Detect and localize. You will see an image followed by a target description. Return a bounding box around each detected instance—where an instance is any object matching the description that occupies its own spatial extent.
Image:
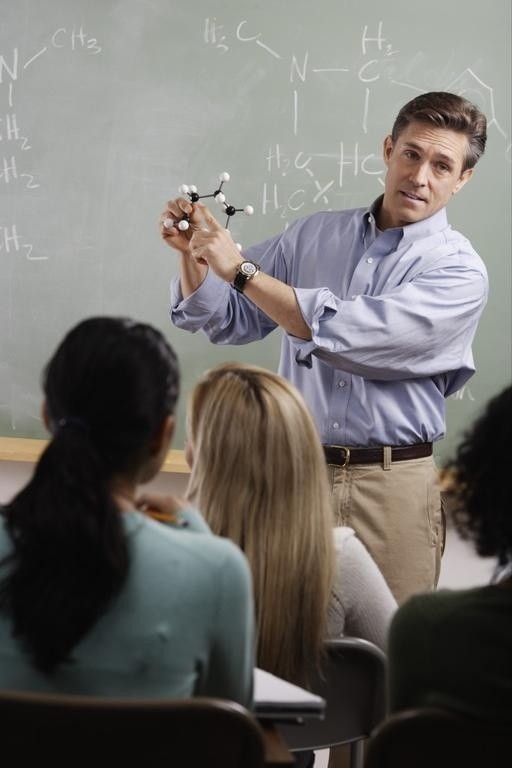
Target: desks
[252,665,327,724]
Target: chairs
[262,634,391,767]
[0,682,269,768]
[359,706,511,767]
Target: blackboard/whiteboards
[0,0,512,474]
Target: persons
[160,92,487,607]
[1,314,255,709]
[185,362,400,692]
[387,382,512,734]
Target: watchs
[233,258,260,293]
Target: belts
[321,442,433,469]
